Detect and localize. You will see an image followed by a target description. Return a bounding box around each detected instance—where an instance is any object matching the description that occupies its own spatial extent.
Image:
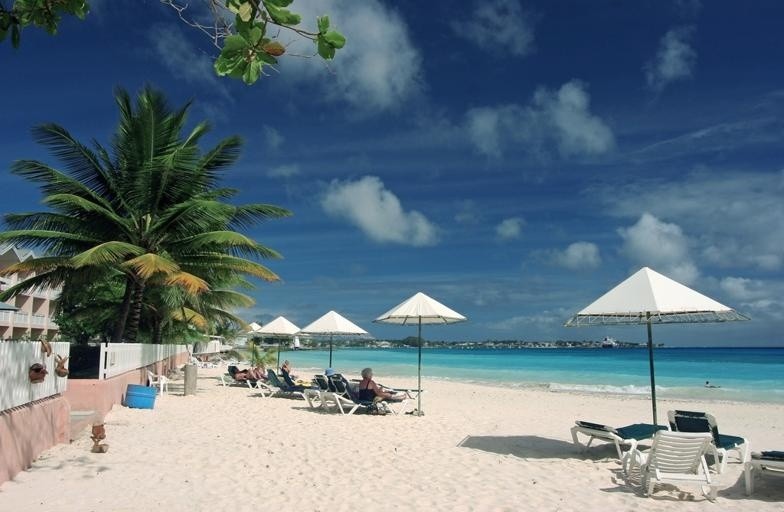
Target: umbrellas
[371,291,467,411]
[250,316,301,375]
[236,321,261,371]
[564,267,752,438]
[299,310,368,367]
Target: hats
[325,369,336,377]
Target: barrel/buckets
[125,384,157,409]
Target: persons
[281,360,290,374]
[231,366,269,382]
[325,368,360,403]
[359,368,406,406]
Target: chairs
[144,353,426,417]
[569,405,784,504]
